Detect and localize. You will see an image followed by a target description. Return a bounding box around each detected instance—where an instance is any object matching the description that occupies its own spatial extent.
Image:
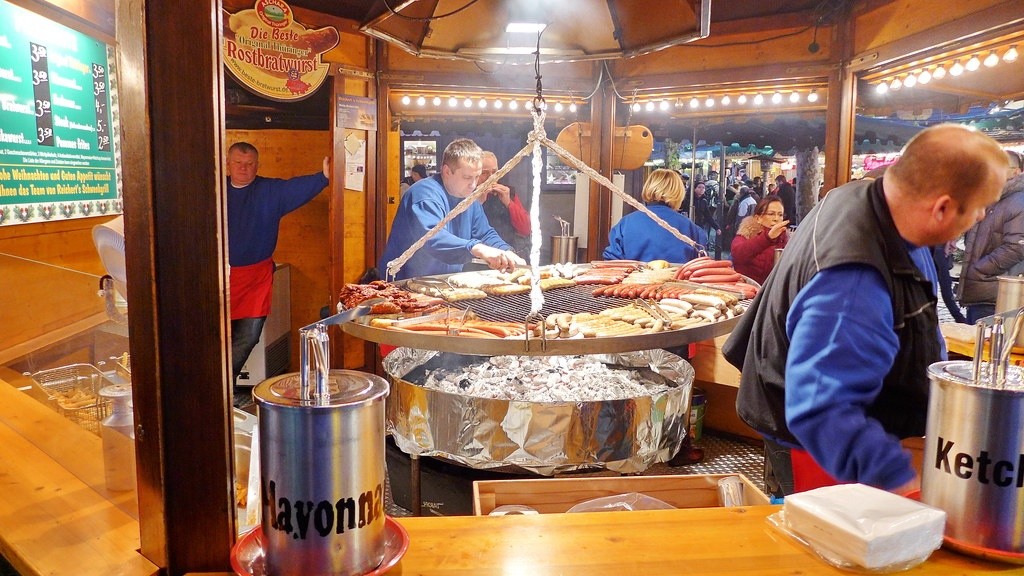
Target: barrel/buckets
[688,386,707,444]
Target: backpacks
[705,184,717,208]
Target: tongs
[446,304,470,337]
[635,297,672,327]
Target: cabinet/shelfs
[547,155,577,185]
[405,152,436,171]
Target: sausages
[369,256,759,340]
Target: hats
[413,166,428,178]
[682,173,689,178]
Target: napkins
[783,483,945,569]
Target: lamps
[505,6,548,35]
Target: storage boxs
[472,474,772,517]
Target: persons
[720,125,1010,502]
[681,173,690,187]
[768,175,795,225]
[404,165,427,186]
[957,149,1024,322]
[381,139,526,281]
[730,195,789,284]
[930,240,963,321]
[680,183,721,236]
[602,169,706,467]
[477,150,529,245]
[695,168,764,249]
[226,142,330,404]
